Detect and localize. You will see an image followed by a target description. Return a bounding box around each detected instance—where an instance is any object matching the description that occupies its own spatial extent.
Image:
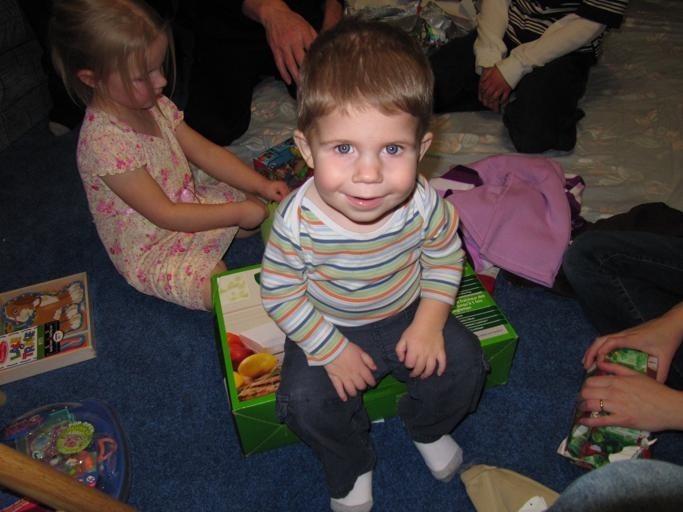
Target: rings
[599,400,606,413]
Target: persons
[340,0,499,119]
[423,0,629,155]
[258,15,492,510]
[151,0,327,150]
[64,0,291,319]
[539,301,683,511]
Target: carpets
[0,119,681,512]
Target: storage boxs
[211,261,520,458]
